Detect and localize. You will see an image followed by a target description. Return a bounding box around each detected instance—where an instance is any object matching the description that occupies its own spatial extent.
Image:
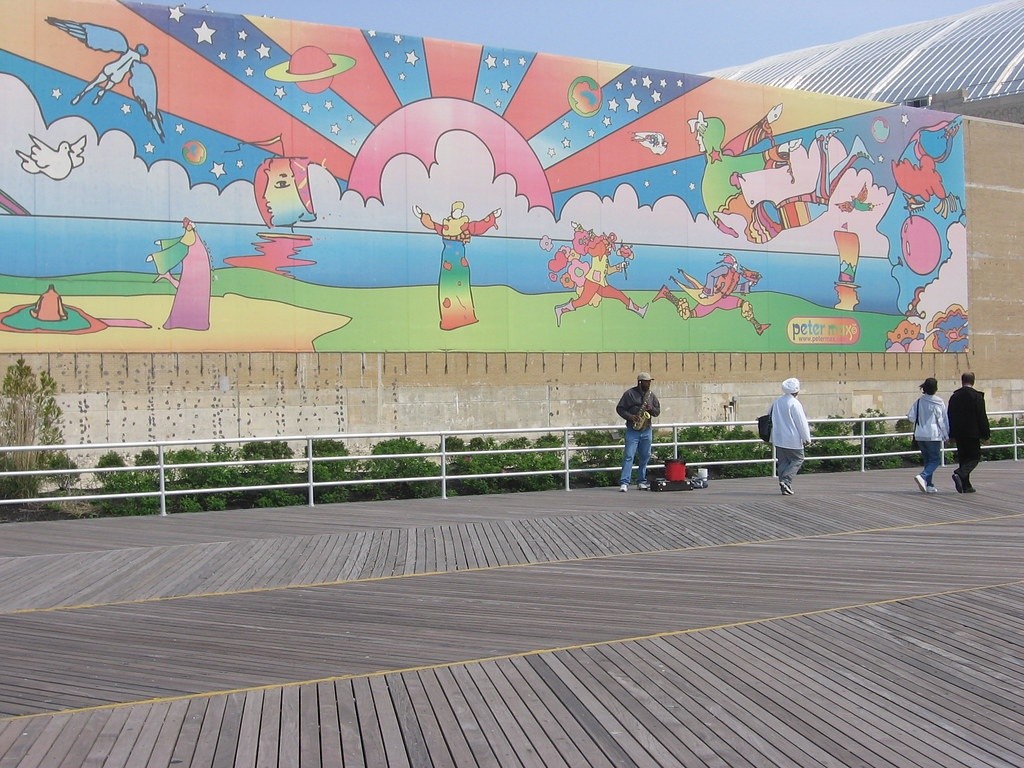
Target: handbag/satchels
[911,434,921,451]
[758,402,773,443]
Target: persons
[616,372,660,492]
[768,378,812,495]
[908,377,950,494]
[947,372,990,493]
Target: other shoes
[779,482,795,495]
[915,475,928,492]
[927,487,937,493]
[620,484,628,492]
[963,487,976,493]
[952,474,963,493]
[639,483,647,489]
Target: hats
[781,378,800,393]
[637,372,655,381]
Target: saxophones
[631,387,652,431]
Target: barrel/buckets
[697,468,709,482]
